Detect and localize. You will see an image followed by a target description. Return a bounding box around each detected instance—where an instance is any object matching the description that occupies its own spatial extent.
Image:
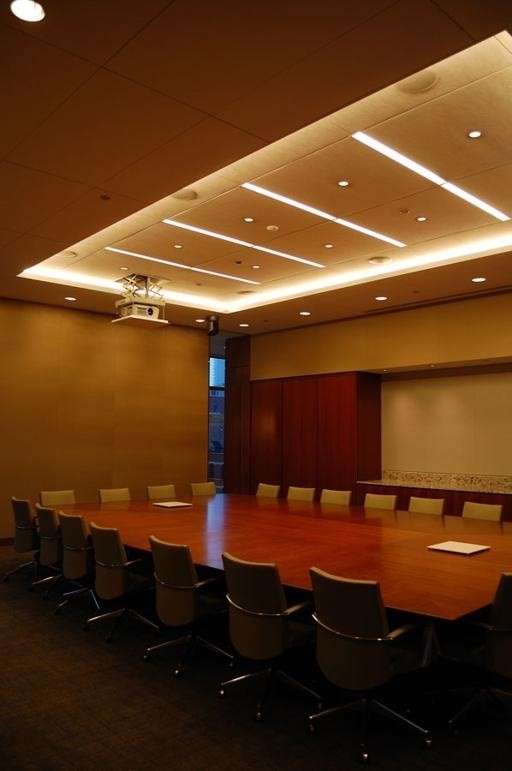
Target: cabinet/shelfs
[319,371,382,504]
[250,378,283,497]
[283,374,319,499]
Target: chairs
[447,573,512,739]
[29,502,63,592]
[53,511,101,615]
[305,567,432,761]
[2,495,41,583]
[143,535,235,678]
[81,522,160,643]
[216,552,324,722]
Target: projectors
[112,298,169,331]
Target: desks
[32,492,512,622]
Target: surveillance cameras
[206,316,219,337]
